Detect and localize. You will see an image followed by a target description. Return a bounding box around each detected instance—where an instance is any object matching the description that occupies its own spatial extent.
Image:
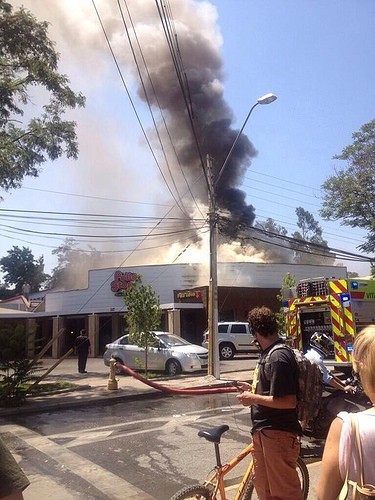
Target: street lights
[205,93,278,379]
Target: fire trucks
[282,276,375,377]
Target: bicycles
[170,425,309,500]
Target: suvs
[201,322,260,360]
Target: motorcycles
[299,375,374,439]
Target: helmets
[309,332,335,358]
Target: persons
[315,325,375,500]
[236,306,304,500]
[22,281,31,302]
[304,332,360,413]
[74,329,92,373]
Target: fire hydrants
[108,355,118,390]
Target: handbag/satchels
[336,411,375,500]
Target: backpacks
[265,343,327,436]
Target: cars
[103,331,209,376]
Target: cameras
[232,380,244,392]
